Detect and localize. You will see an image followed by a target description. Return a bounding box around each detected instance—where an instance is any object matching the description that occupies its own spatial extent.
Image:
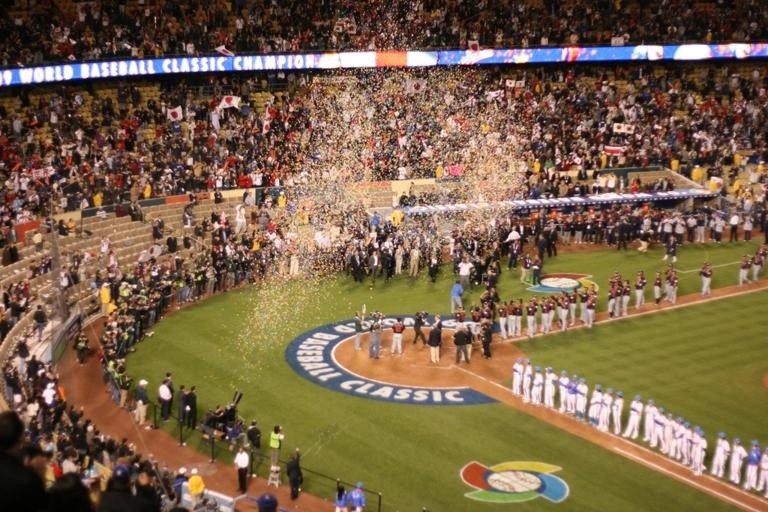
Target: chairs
[1,2,766,417]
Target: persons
[0,2,768,512]
